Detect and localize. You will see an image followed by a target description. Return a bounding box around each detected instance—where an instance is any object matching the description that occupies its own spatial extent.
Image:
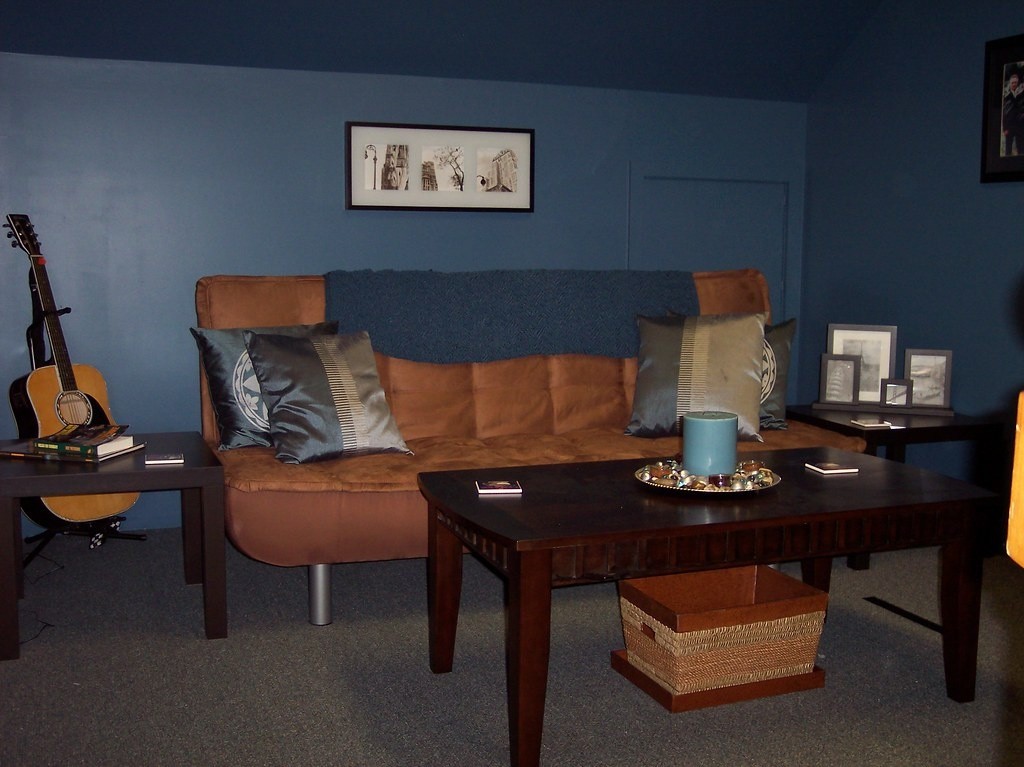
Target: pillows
[624,314,766,445]
[242,329,415,465]
[755,318,803,430]
[189,321,341,451]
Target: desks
[415,446,999,766]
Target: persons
[1002,74,1024,156]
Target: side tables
[0,431,230,661]
[785,402,998,570]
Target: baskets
[619,566,829,693]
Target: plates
[634,467,781,492]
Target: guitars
[3,212,139,533]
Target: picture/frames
[880,377,913,408]
[344,121,535,213]
[980,36,1024,183]
[826,323,898,404]
[903,348,952,409]
[818,353,861,405]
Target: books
[0,422,185,465]
[805,461,860,474]
[475,480,523,494]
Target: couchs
[193,265,869,626]
